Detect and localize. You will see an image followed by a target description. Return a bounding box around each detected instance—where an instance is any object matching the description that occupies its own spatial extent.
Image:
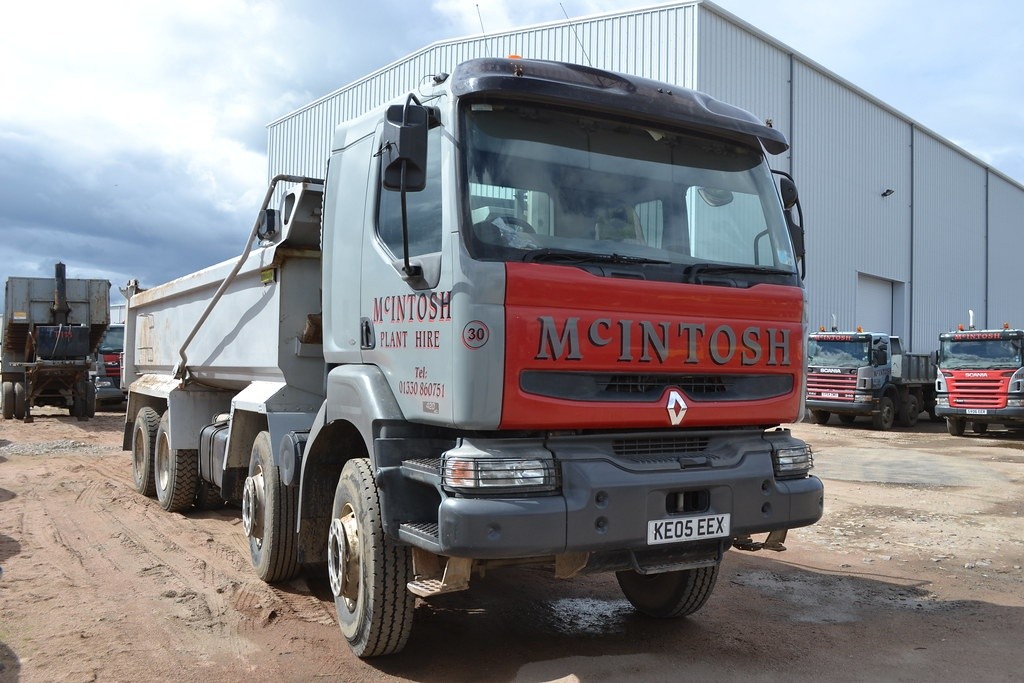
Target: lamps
[881,189,894,197]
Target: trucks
[91,323,132,410]
[120,56,825,660]
[931,309,1024,436]
[805,326,939,430]
